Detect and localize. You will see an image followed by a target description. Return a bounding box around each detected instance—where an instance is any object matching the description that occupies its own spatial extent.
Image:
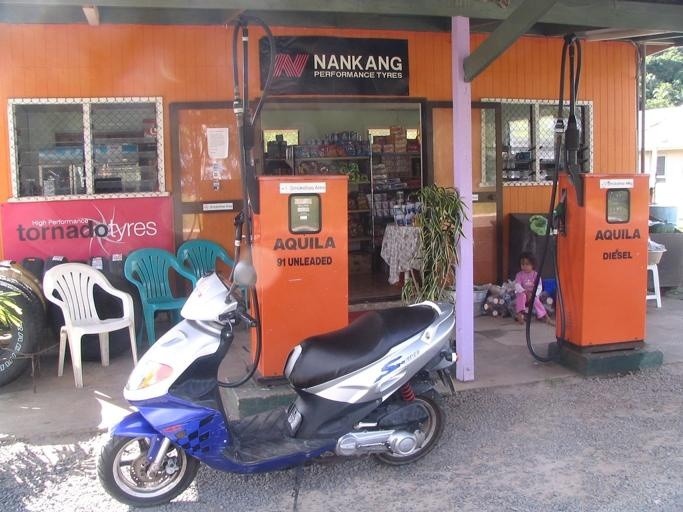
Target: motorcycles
[98,260,456,507]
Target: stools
[646,264,661,308]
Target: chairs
[124,248,197,353]
[42,262,138,388]
[176,237,248,322]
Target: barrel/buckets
[649,205,677,228]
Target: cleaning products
[393,202,420,226]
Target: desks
[509,213,555,282]
[380,223,424,285]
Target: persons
[507,253,555,327]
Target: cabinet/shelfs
[265,133,421,254]
